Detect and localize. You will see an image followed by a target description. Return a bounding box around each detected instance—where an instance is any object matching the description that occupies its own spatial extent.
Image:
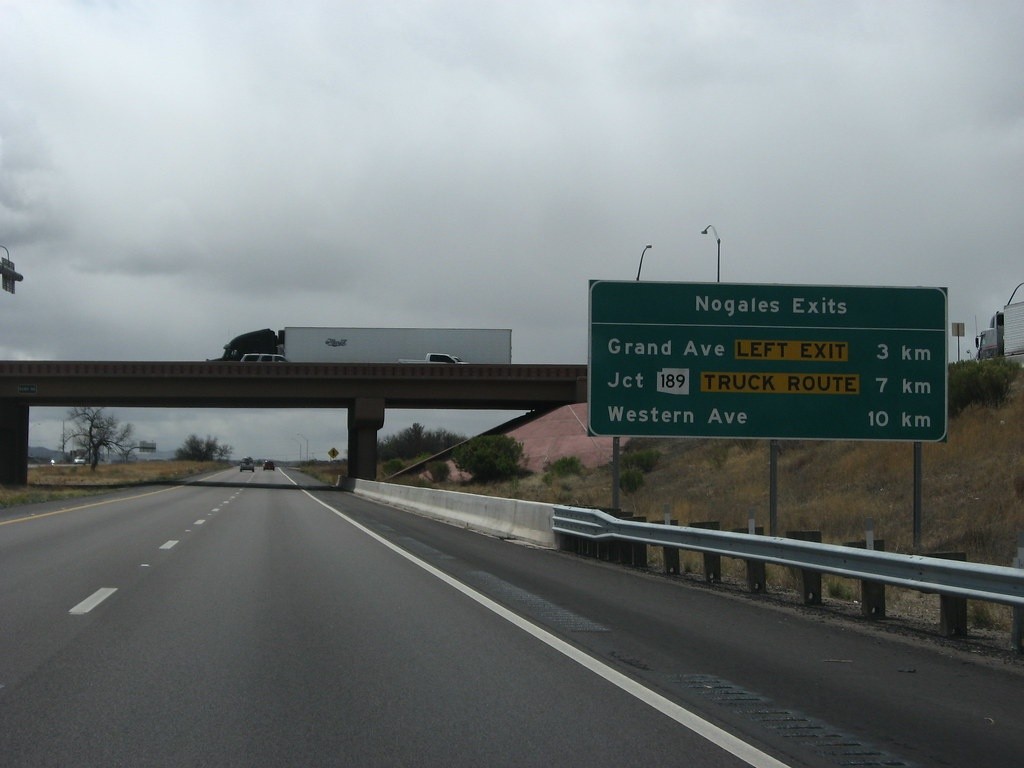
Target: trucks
[975,302,1024,358]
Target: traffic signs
[583,280,947,442]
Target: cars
[263,459,274,471]
[240,456,255,473]
[75,456,86,464]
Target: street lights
[292,438,301,460]
[297,433,308,461]
[610,245,653,282]
[702,225,720,281]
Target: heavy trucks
[202,328,513,364]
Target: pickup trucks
[398,352,470,364]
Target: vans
[241,353,289,363]
